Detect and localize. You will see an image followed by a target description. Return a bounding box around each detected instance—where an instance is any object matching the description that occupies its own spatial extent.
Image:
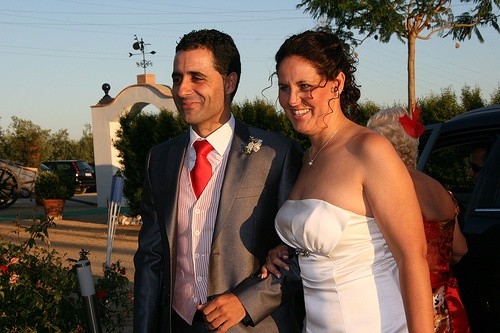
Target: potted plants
[33,173,76,220]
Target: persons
[261,31,434,333]
[366,102,471,333]
[133,29,304,333]
[467,140,494,185]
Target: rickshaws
[0,159,98,211]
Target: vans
[42,160,96,194]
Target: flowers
[245,136,262,152]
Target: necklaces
[308,118,347,166]
